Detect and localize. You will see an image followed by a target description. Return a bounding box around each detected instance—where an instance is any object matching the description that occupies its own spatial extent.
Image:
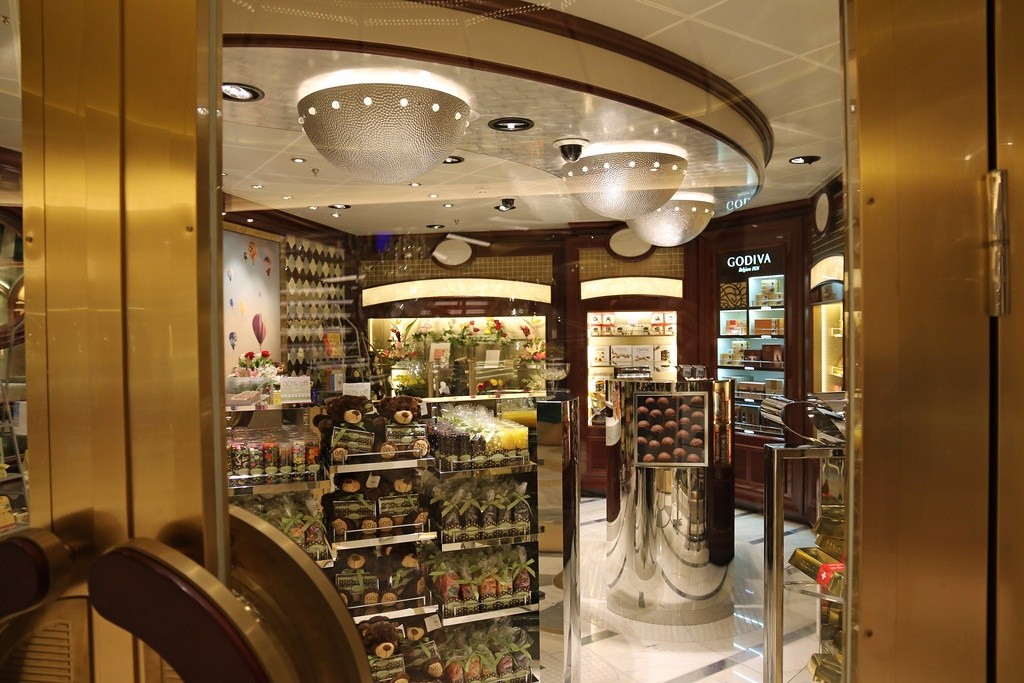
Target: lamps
[556,139,689,220]
[431,238,475,270]
[625,189,716,247]
[810,186,835,237]
[293,68,474,186]
[604,225,657,263]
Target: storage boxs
[726,279,784,426]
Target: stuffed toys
[396,613,444,683]
[373,469,429,532]
[332,547,380,609]
[357,616,410,683]
[377,543,427,607]
[317,395,378,462]
[374,393,431,457]
[321,471,379,534]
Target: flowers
[412,320,432,341]
[488,319,509,345]
[518,319,548,365]
[440,319,457,342]
[457,320,480,345]
[388,318,416,352]
[238,350,271,370]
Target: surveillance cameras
[501,198,514,208]
[553,138,590,163]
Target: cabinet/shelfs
[699,246,803,527]
[221,278,682,682]
